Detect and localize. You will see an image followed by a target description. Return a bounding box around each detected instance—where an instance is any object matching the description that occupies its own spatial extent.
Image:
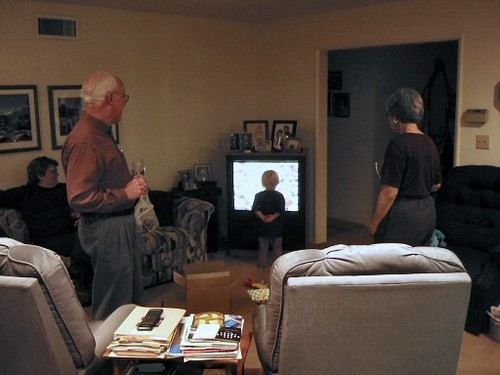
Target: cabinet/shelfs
[174,187,221,254]
[225,212,306,256]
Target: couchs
[374,164,498,336]
[252,243,472,375]
[0,189,214,305]
[1,237,137,375]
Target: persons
[0,157,95,307]
[62,73,149,320]
[368,88,442,246]
[253,170,285,270]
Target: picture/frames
[193,164,211,183]
[179,170,192,189]
[271,120,297,153]
[333,92,351,118]
[239,132,253,151]
[328,91,334,116]
[328,70,342,90]
[47,85,120,150]
[0,85,42,154]
[243,120,269,152]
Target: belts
[80,208,134,218]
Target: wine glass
[374,158,385,180]
[130,158,149,201]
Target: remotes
[137,309,162,331]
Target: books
[106,307,186,359]
[166,311,244,363]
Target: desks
[102,320,254,375]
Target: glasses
[114,95,129,102]
[48,169,58,174]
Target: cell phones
[224,319,239,328]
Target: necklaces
[401,128,421,132]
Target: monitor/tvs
[228,154,306,219]
